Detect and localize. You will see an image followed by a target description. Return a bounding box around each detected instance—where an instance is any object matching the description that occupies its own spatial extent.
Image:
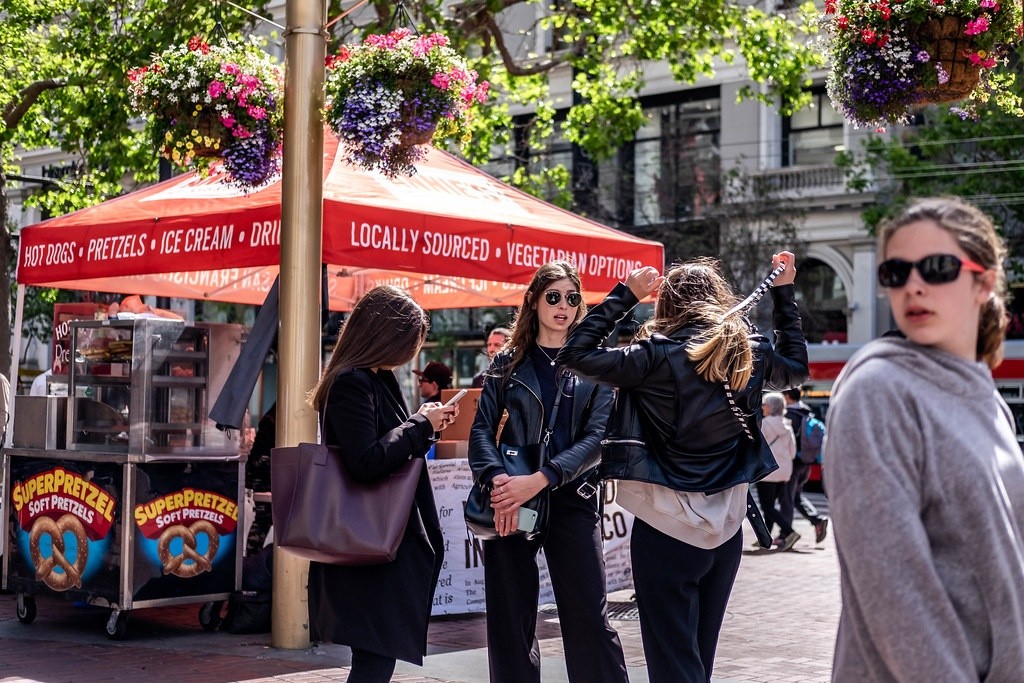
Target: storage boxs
[435,441,470,459]
[193,322,243,450]
[441,388,504,440]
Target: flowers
[318,27,490,180]
[127,39,284,199]
[808,0,1024,133]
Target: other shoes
[814,519,828,543]
[780,532,801,551]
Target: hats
[412,362,452,387]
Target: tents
[2,106,666,586]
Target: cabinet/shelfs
[65,319,212,450]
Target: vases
[178,111,229,158]
[906,15,981,105]
[378,96,437,148]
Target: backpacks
[790,410,825,464]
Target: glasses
[542,292,581,307]
[419,378,430,384]
[878,254,985,288]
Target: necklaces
[535,337,560,366]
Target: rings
[499,520,505,522]
[499,486,503,500]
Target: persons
[245,401,274,556]
[30,369,53,395]
[823,200,1024,683]
[471,329,511,388]
[467,261,629,683]
[561,250,811,683]
[758,387,828,551]
[0,351,10,458]
[309,286,460,683]
[411,362,453,442]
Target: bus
[786,340,1024,487]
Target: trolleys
[5,316,244,638]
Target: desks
[5,447,249,639]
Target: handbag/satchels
[465,442,549,547]
[269,369,424,565]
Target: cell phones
[493,507,538,533]
[444,389,467,407]
